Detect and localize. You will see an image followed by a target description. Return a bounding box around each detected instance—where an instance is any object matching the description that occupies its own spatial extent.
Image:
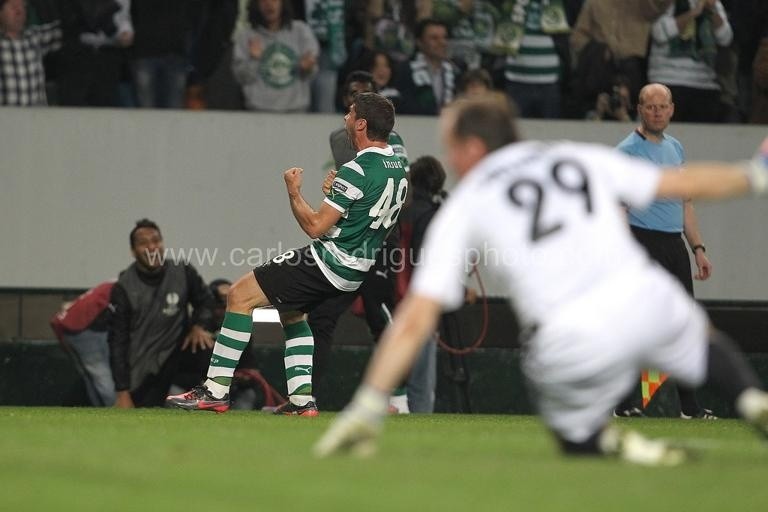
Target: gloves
[741,136,767,197]
[313,386,389,461]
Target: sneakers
[680,408,719,420]
[733,387,768,436]
[165,382,231,413]
[597,427,676,466]
[613,404,647,417]
[261,400,319,416]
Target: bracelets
[692,242,707,255]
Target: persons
[161,90,408,416]
[355,153,449,414]
[609,81,719,419]
[306,71,411,416]
[305,83,768,470]
[107,216,219,408]
[51,273,123,407]
[208,278,285,410]
[0,0,768,123]
